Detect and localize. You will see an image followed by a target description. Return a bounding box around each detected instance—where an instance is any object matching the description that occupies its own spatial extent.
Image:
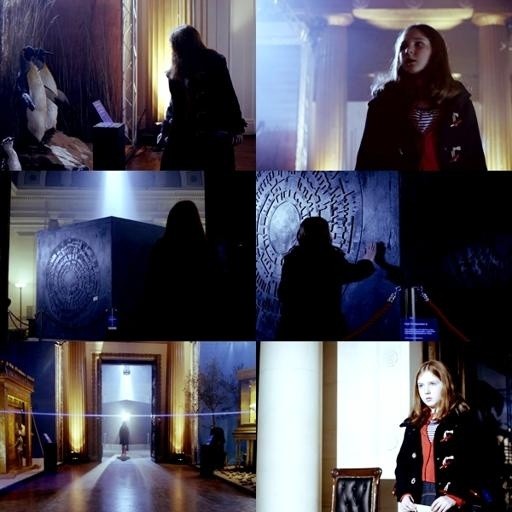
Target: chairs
[331,467,381,512]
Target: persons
[353,23,488,171]
[152,197,215,340]
[273,215,378,340]
[391,360,480,512]
[120,422,130,453]
[156,26,248,172]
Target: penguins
[1,135,23,171]
[14,45,70,143]
